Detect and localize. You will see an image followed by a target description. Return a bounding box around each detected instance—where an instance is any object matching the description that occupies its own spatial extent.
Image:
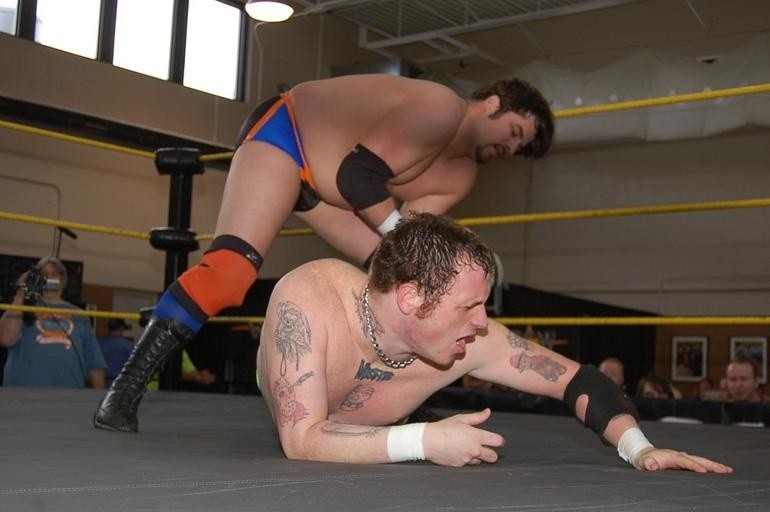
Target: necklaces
[363,288,418,369]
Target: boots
[94,314,195,432]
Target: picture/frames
[669,333,767,388]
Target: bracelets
[387,422,427,462]
[617,428,654,465]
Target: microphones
[57,225,78,240]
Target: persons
[0,257,108,389]
[93,74,556,433]
[145,348,217,390]
[97,318,135,389]
[256,210,733,474]
[598,356,770,403]
